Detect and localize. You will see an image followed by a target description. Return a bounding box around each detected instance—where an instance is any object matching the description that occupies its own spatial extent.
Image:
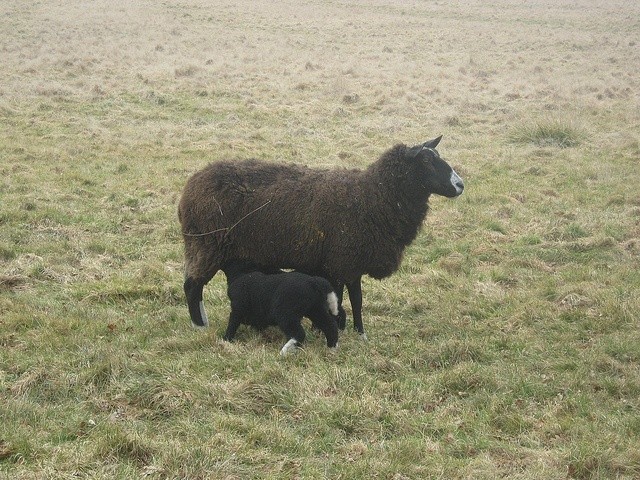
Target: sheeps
[177,134,466,329]
[221,268,340,358]
